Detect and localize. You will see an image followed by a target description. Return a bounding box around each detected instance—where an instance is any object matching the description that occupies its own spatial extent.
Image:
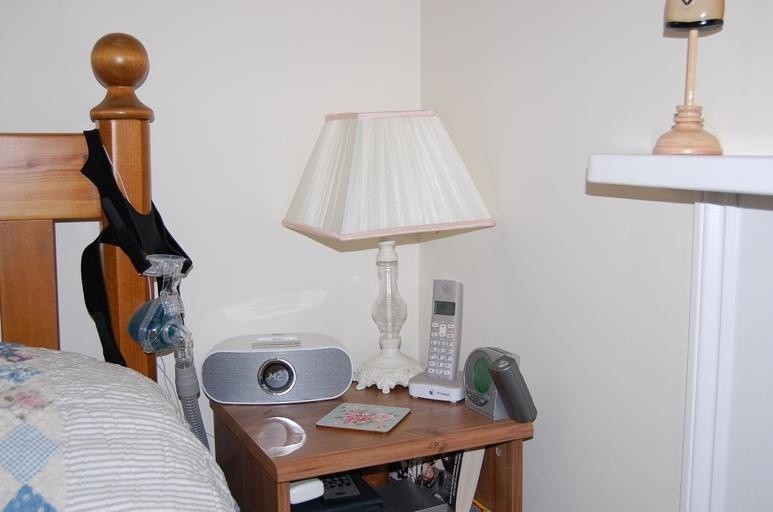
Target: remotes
[320,473,361,503]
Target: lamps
[280,110,495,395]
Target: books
[386,448,485,512]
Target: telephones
[409,280,465,404]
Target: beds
[0,31,239,511]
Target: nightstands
[209,381,534,511]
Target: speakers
[200,334,353,405]
